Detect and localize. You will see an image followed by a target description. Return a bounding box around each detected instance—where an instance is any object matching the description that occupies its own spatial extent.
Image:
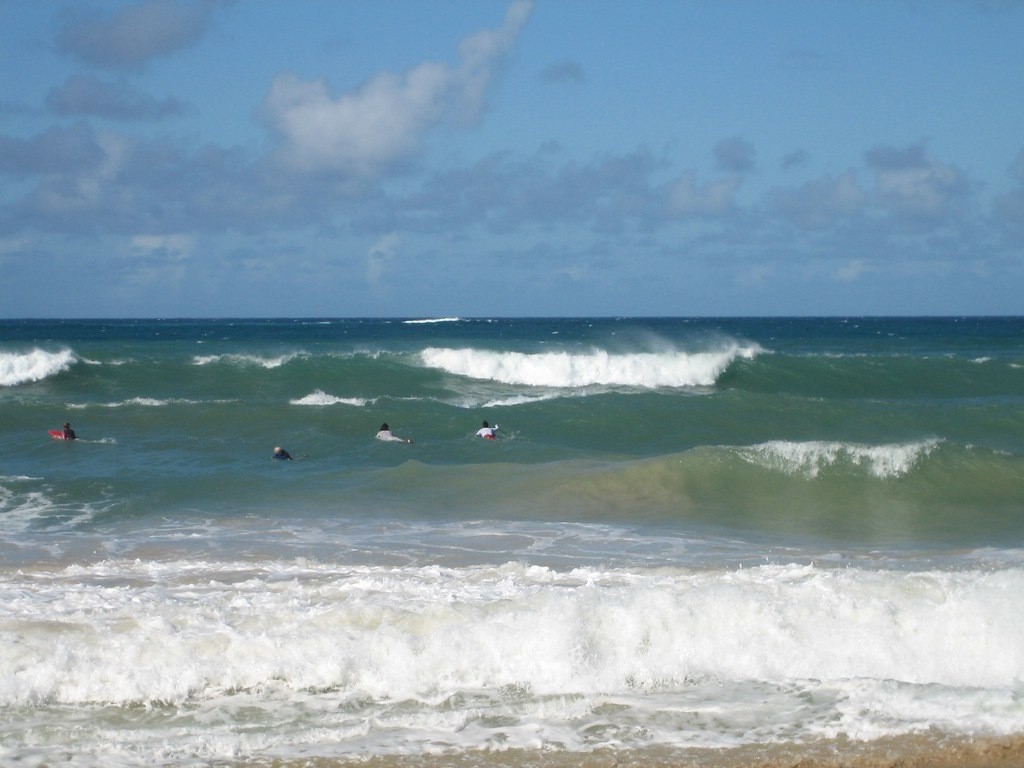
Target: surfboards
[48,430,65,439]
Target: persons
[475,420,499,439]
[377,423,405,441]
[272,446,293,461]
[54,422,76,440]
